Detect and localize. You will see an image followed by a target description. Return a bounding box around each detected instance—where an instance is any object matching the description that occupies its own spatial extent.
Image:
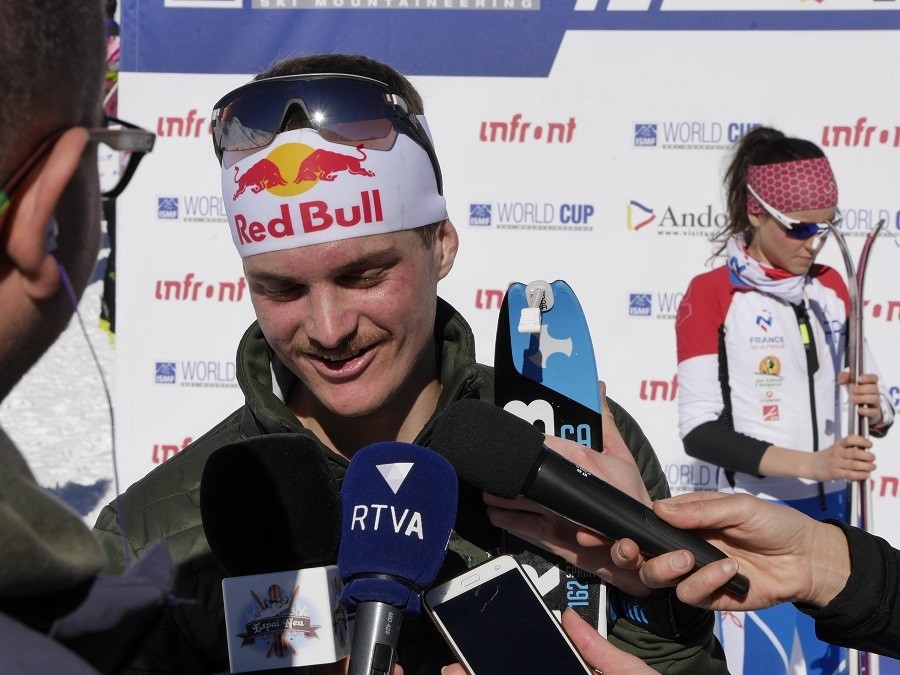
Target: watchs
[878,397,894,426]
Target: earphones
[43,214,61,253]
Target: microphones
[198,432,353,675]
[433,397,752,594]
[335,440,458,675]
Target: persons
[91,54,900,675]
[0,0,157,675]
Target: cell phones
[421,554,593,675]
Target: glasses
[0,116,155,209]
[211,73,443,196]
[748,183,842,239]
